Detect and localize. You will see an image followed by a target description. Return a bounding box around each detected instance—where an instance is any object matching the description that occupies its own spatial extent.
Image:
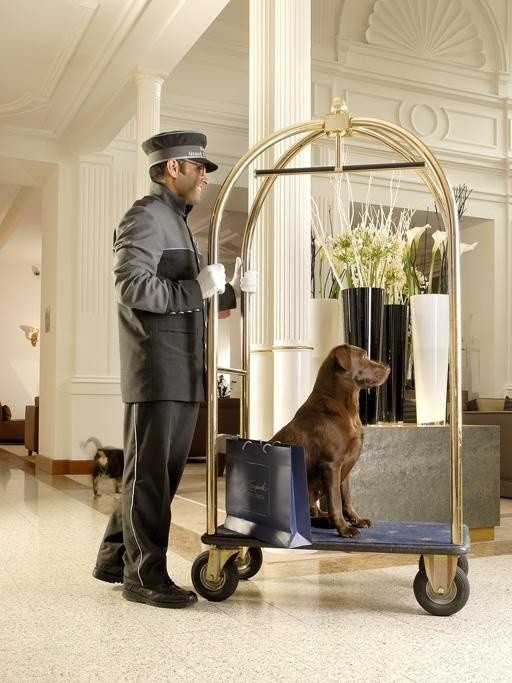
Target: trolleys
[190,95,471,617]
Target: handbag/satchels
[223,436,313,548]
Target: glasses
[178,159,207,175]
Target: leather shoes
[121,580,198,610]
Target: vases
[408,293,449,424]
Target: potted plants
[312,169,408,425]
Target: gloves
[229,255,260,301]
[196,263,227,300]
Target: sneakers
[91,565,125,585]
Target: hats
[141,129,219,174]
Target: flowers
[403,224,478,295]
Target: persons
[92,131,258,611]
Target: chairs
[0,404,24,444]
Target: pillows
[1,404,11,420]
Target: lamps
[19,324,39,347]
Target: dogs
[83,435,125,498]
[268,342,392,538]
